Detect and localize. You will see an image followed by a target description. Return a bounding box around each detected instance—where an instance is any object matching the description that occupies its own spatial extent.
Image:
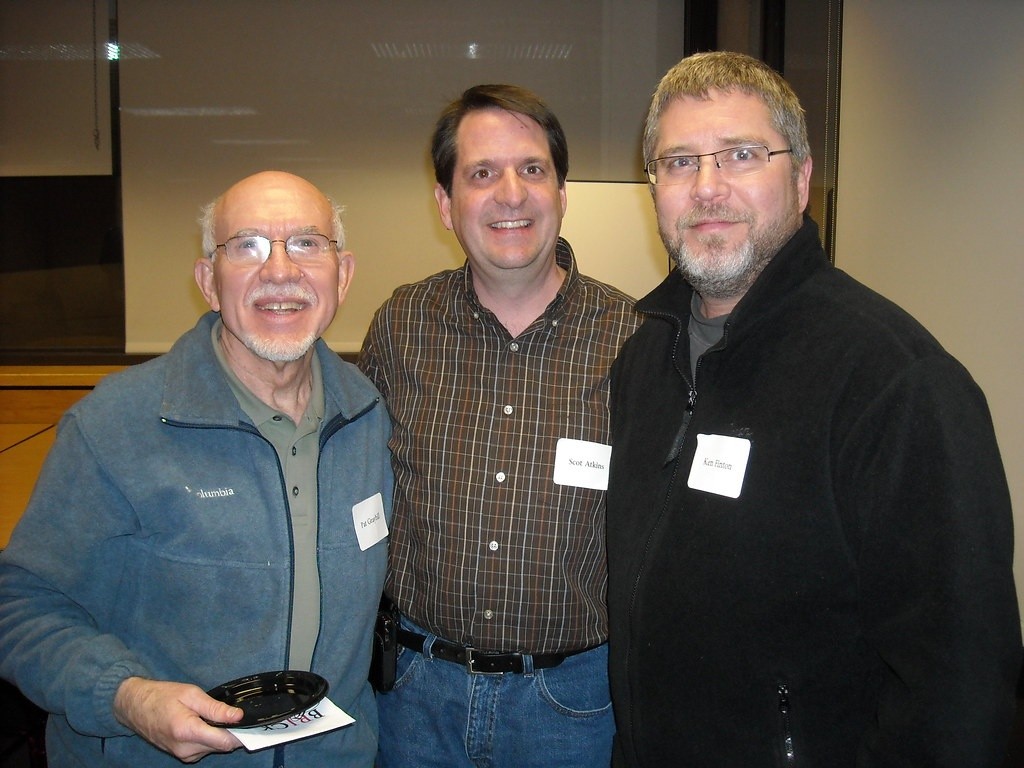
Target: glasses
[209,233,339,264]
[645,145,793,187]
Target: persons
[606,50,1024,768]
[0,169,395,768]
[353,83,643,768]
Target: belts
[395,628,608,676]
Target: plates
[199,671,329,730]
